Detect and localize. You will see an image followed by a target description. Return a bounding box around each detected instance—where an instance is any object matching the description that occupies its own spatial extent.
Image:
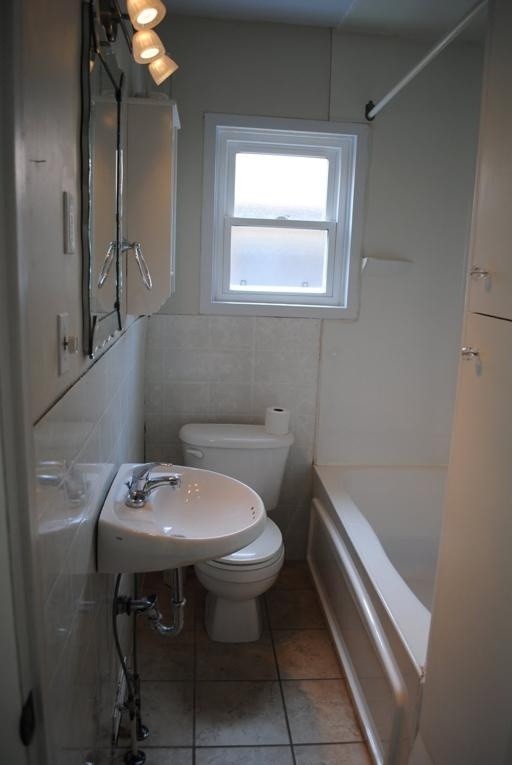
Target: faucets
[34,460,89,505]
[126,461,183,507]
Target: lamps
[98,1,180,86]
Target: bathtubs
[307,464,446,764]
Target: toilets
[179,421,295,643]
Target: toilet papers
[266,406,290,437]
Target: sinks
[96,462,267,573]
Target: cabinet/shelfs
[124,93,189,318]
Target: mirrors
[79,0,129,362]
